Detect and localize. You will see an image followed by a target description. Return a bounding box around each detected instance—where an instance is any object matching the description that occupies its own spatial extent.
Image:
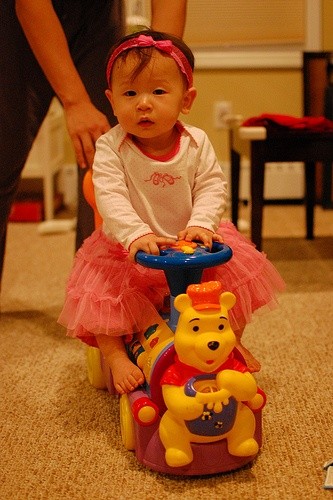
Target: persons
[57,29,287,394]
[0,0,187,291]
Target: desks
[21,115,66,221]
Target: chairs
[228,51,333,254]
